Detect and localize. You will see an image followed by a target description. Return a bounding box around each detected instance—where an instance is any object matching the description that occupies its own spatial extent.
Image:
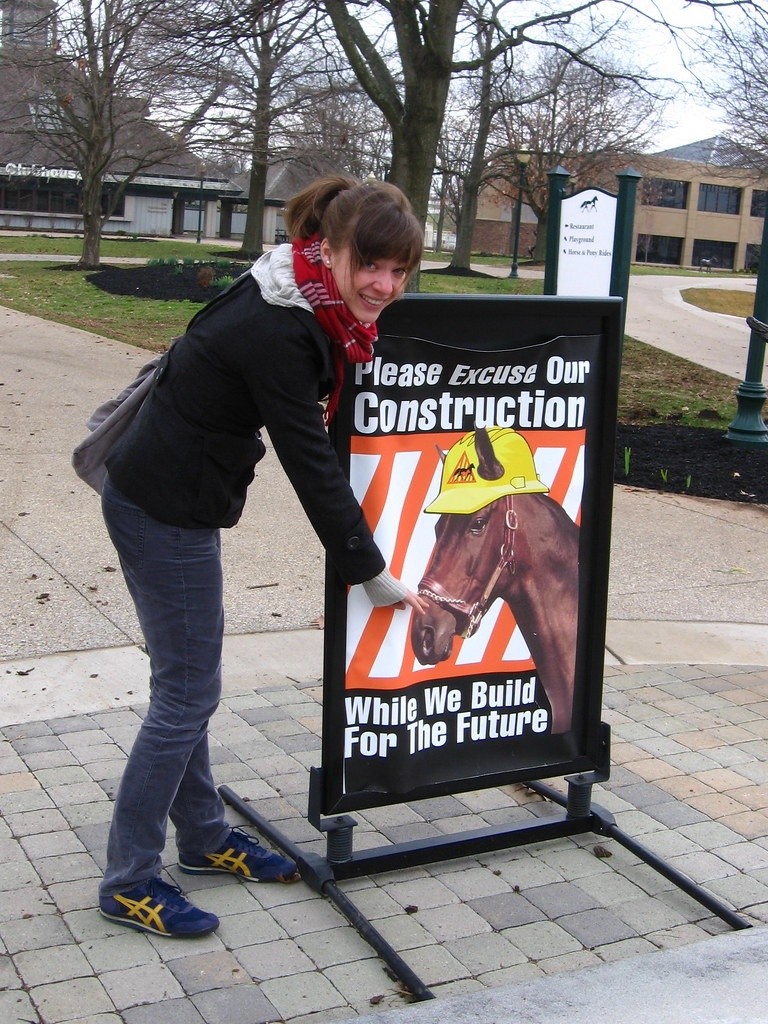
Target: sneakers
[99,878,220,938]
[178,827,297,883]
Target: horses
[412,427,579,735]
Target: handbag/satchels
[71,356,166,497]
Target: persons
[72,173,429,942]
